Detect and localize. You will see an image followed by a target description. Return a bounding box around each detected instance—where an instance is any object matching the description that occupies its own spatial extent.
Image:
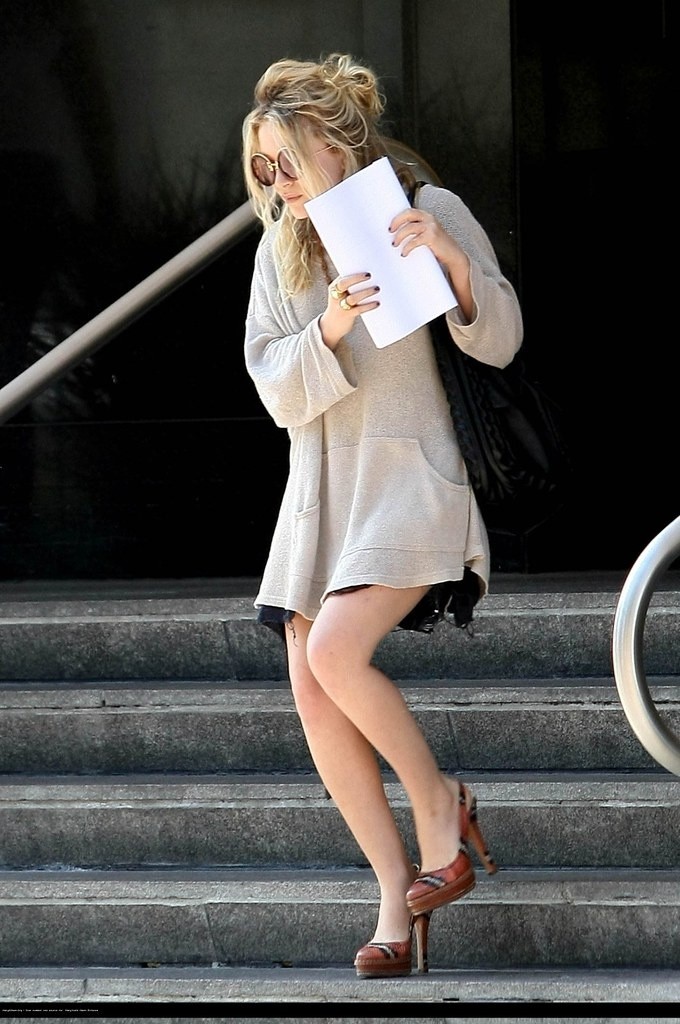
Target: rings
[331,285,344,299]
[340,299,351,310]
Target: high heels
[355,864,433,977]
[405,780,498,916]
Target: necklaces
[317,242,333,284]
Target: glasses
[252,144,334,187]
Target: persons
[240,52,524,977]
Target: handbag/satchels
[408,180,577,536]
